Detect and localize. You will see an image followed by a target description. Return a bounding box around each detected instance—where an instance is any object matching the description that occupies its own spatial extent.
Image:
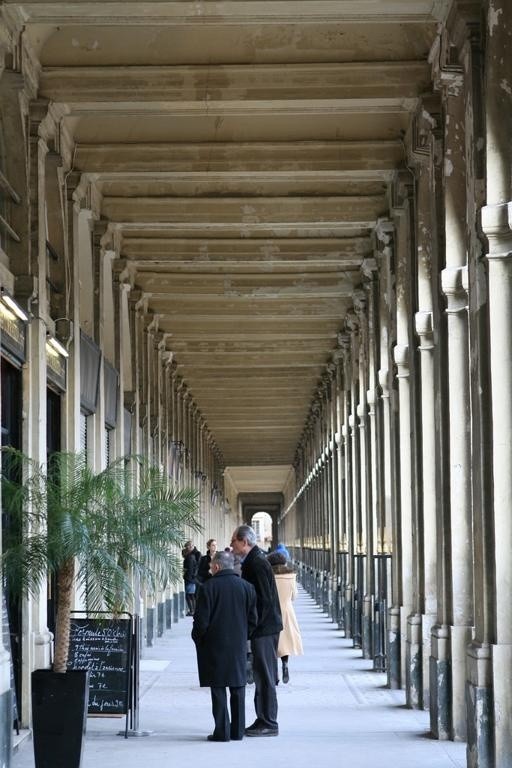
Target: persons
[181,540,294,618]
[228,526,283,737]
[191,551,258,742]
[268,552,304,683]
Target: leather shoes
[283,662,289,683]
[208,735,231,742]
[245,723,278,736]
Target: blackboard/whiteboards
[67,610,132,714]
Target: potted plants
[2,443,209,767]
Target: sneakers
[186,611,195,616]
